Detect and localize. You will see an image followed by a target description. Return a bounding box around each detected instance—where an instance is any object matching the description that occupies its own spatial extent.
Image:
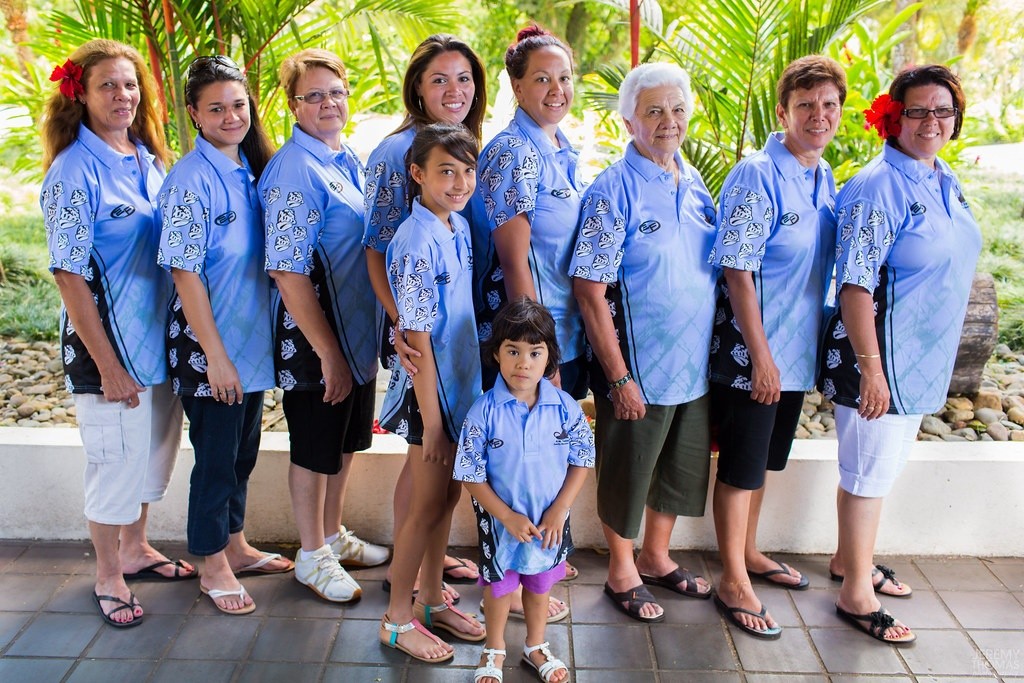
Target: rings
[867,406,874,409]
[218,391,225,394]
[227,389,236,396]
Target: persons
[377,121,486,663]
[467,21,588,626]
[362,36,480,603]
[452,293,595,683]
[156,56,296,616]
[706,53,846,639]
[40,38,198,629]
[256,47,390,602]
[567,62,719,622]
[818,65,981,645]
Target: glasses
[293,90,350,105]
[185,55,241,81]
[901,108,959,119]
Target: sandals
[380,613,454,662]
[413,599,486,640]
[473,643,506,683]
[522,636,570,683]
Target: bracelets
[855,353,880,358]
[607,371,632,389]
[394,315,399,326]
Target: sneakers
[330,525,389,566]
[294,548,361,601]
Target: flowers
[49,58,85,104]
[863,94,905,140]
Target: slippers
[382,578,460,606]
[747,561,809,588]
[442,554,478,584]
[834,600,916,642]
[92,590,143,627]
[231,551,294,574]
[200,584,255,614]
[123,560,198,582]
[604,581,665,623]
[639,565,712,599]
[830,565,912,596]
[480,596,569,622]
[560,566,578,580]
[713,591,782,638]
